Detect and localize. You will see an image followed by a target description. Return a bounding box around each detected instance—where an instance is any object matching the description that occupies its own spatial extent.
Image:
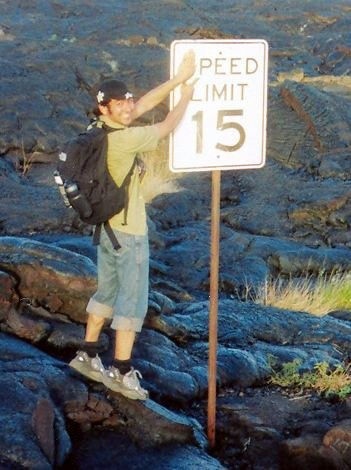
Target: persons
[68,49,200,401]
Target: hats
[96,80,135,105]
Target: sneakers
[68,349,105,383]
[101,365,149,401]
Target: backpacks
[53,116,137,250]
